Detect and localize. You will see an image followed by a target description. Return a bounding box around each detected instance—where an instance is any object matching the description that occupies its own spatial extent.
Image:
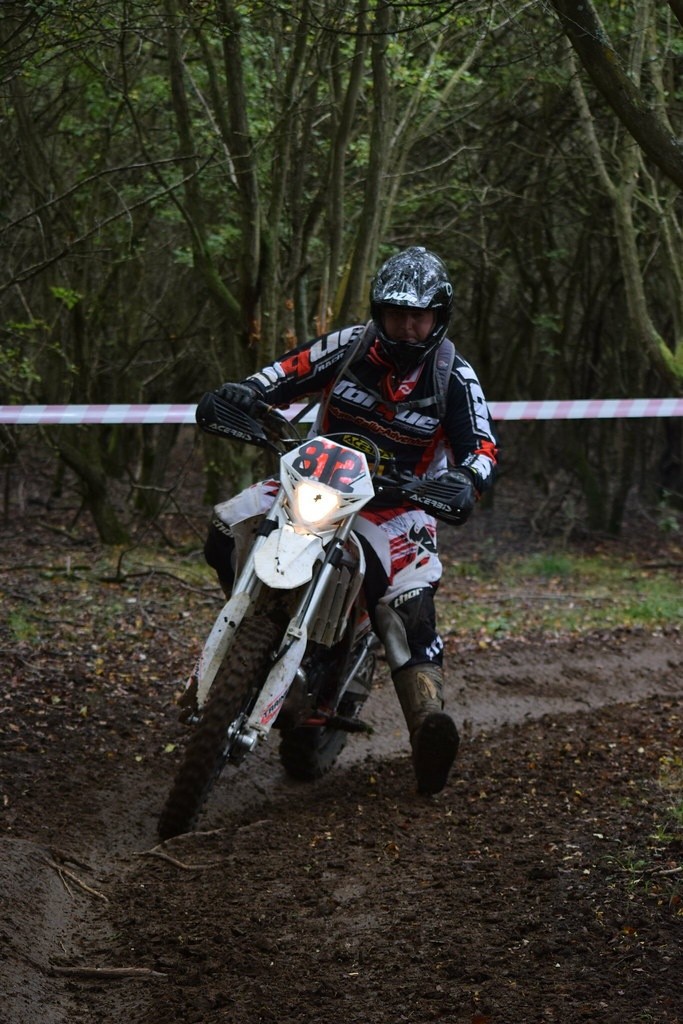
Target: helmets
[367,244,453,369]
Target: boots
[389,662,458,794]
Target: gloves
[217,380,256,408]
[432,468,475,501]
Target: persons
[203,245,498,795]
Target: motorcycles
[156,389,469,847]
